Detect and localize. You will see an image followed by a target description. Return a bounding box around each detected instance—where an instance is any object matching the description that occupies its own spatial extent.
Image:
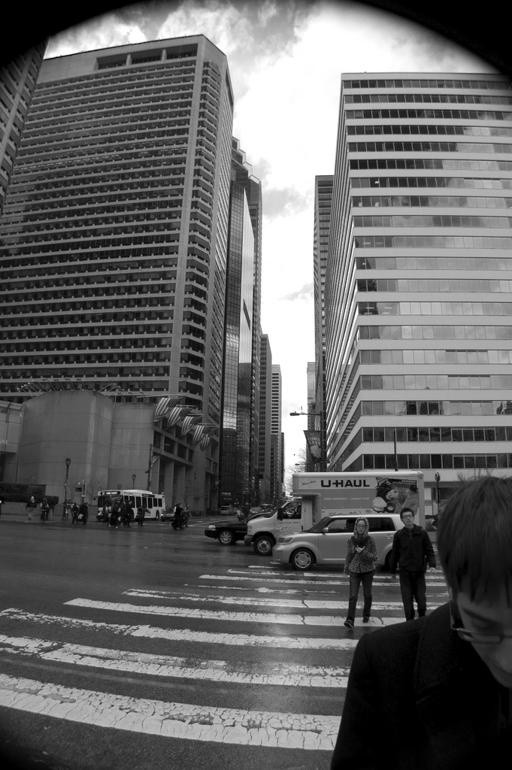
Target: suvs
[271,513,405,574]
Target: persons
[329,474,512,770]
[389,507,437,622]
[342,516,378,630]
[237,500,301,522]
[23,494,191,532]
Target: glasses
[446,587,511,645]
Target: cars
[160,505,190,522]
[204,512,274,546]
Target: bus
[92,489,166,522]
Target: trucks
[244,469,426,556]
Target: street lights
[62,458,71,520]
[434,472,440,516]
[289,410,327,472]
[131,474,136,489]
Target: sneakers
[343,620,354,632]
[363,616,369,623]
